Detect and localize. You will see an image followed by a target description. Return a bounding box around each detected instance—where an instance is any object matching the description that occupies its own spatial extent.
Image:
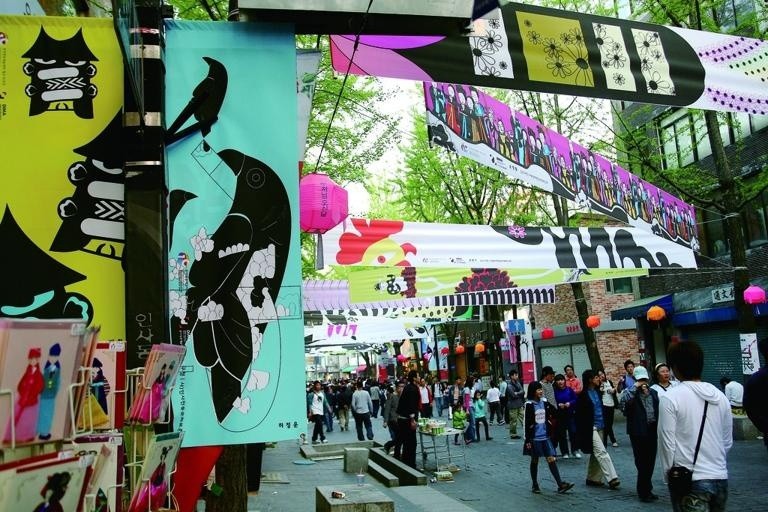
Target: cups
[356,475,365,486]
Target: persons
[303,365,582,494]
[743,338,767,443]
[649,361,678,399]
[595,367,618,448]
[617,359,636,393]
[721,375,744,410]
[646,340,733,512]
[618,366,659,504]
[572,368,620,488]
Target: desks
[316,484,394,512]
[416,426,467,472]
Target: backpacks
[381,391,386,403]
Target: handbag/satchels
[499,396,508,406]
[666,466,693,498]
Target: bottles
[331,491,345,499]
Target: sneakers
[609,479,620,488]
[497,421,505,425]
[383,448,389,454]
[558,481,574,494]
[562,450,582,459]
[639,491,659,503]
[455,437,493,444]
[532,486,541,493]
[612,442,617,447]
[489,422,493,425]
[510,435,521,439]
[302,439,328,444]
[586,479,604,486]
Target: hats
[633,365,649,381]
[541,366,557,376]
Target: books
[0,318,187,512]
